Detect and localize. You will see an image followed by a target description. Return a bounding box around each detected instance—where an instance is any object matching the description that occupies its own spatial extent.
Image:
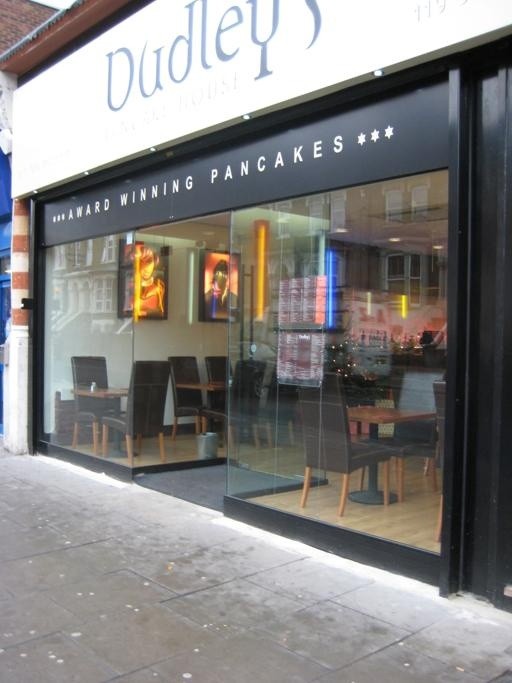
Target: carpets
[131,461,306,512]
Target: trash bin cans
[197,432,218,460]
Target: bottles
[229,374,233,385]
[91,381,98,393]
[359,328,420,350]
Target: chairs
[202,360,268,460]
[299,372,393,518]
[168,355,211,442]
[350,352,444,500]
[100,358,172,468]
[258,360,301,450]
[204,356,234,432]
[69,354,122,459]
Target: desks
[347,406,441,505]
[72,387,139,459]
[174,382,232,449]
